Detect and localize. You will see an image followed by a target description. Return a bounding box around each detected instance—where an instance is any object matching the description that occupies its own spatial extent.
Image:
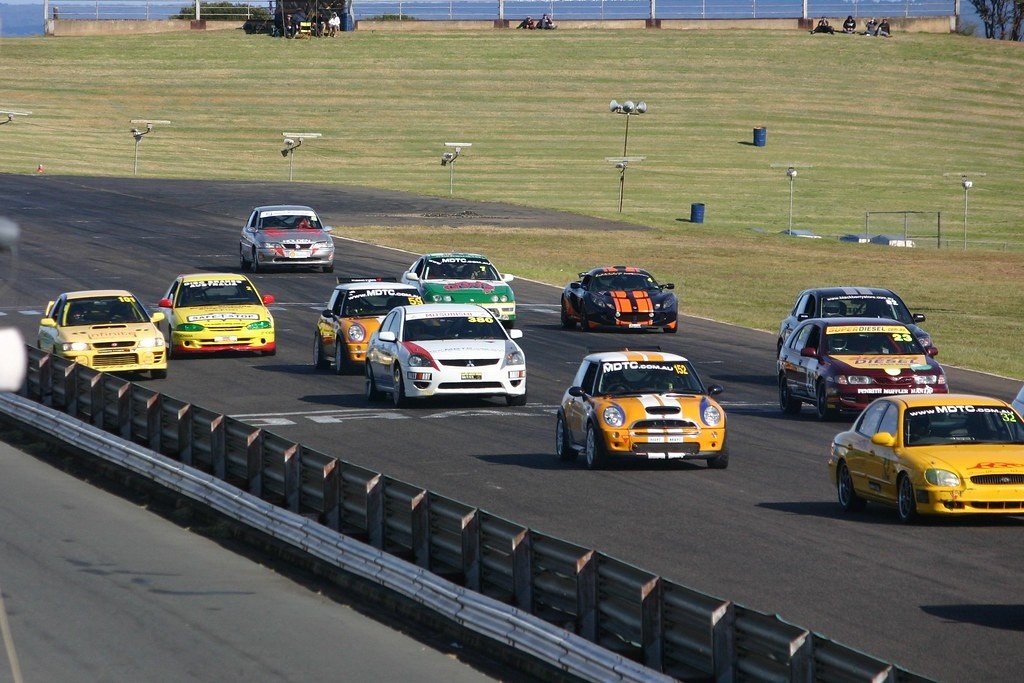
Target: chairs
[650,373,685,389]
[292,218,305,228]
[963,412,992,435]
[348,297,374,309]
[609,277,625,290]
[909,413,932,441]
[463,265,473,278]
[868,301,896,320]
[387,296,410,310]
[263,217,280,229]
[66,304,95,324]
[839,302,847,317]
[300,22,312,40]
[601,370,641,392]
[107,302,133,322]
[189,287,208,305]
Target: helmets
[828,335,848,353]
[825,300,841,316]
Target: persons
[68,303,84,324]
[822,300,844,317]
[411,320,425,339]
[906,411,934,442]
[606,372,624,393]
[285,11,340,37]
[811,16,893,38]
[827,334,852,353]
[183,288,203,306]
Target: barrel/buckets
[754,126,765,146]
[691,203,705,223]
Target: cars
[312,282,428,374]
[556,351,729,470]
[37,288,167,379]
[240,205,335,272]
[157,272,275,356]
[562,265,679,332]
[400,253,516,327]
[777,287,933,360]
[829,394,1024,523]
[777,317,949,421]
[365,304,527,407]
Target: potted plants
[246,7,276,34]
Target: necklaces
[516,13,557,30]
